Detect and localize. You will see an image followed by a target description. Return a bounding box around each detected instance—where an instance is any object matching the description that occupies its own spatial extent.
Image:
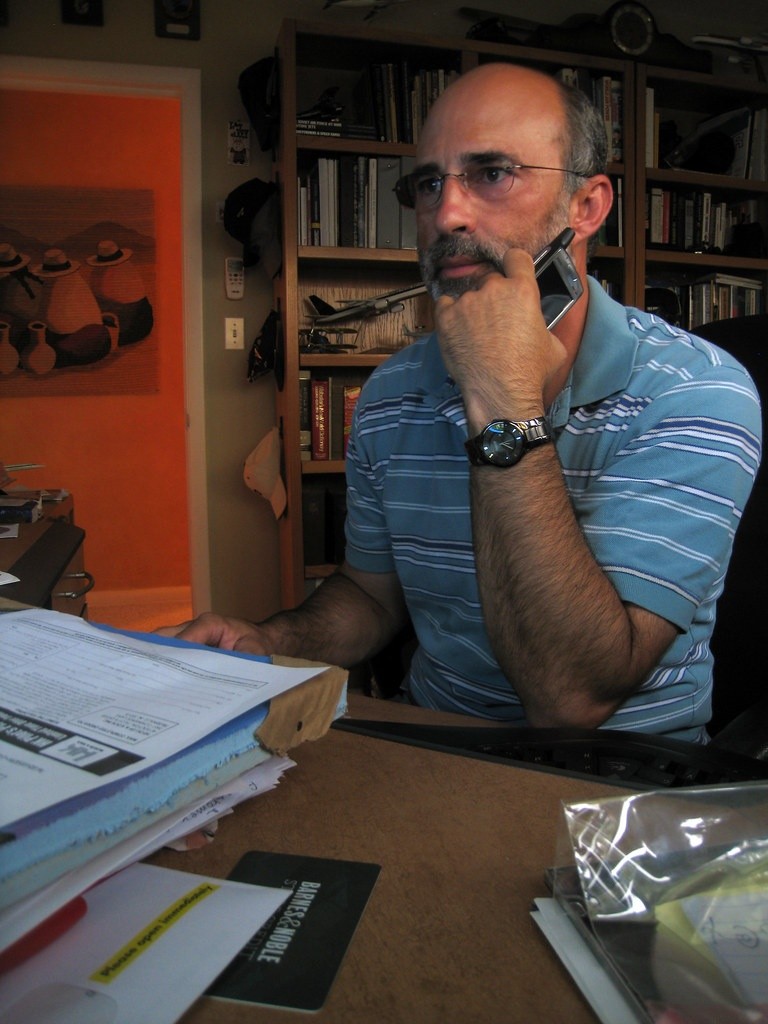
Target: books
[645,187,768,266]
[296,156,418,250]
[299,369,364,460]
[380,62,459,144]
[593,178,622,248]
[294,116,377,142]
[302,484,347,564]
[646,272,768,338]
[555,69,622,162]
[592,269,614,297]
[646,87,768,183]
[0,604,352,952]
[296,275,433,354]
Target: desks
[138,692,768,1024]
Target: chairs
[690,313,768,767]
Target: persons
[149,57,763,744]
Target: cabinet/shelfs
[269,17,768,612]
[0,487,94,623]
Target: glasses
[392,156,590,210]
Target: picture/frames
[0,56,212,623]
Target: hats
[237,46,279,163]
[248,297,285,392]
[224,172,280,268]
[242,416,287,520]
[250,192,281,280]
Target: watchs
[465,413,555,468]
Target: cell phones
[533,228,583,330]
